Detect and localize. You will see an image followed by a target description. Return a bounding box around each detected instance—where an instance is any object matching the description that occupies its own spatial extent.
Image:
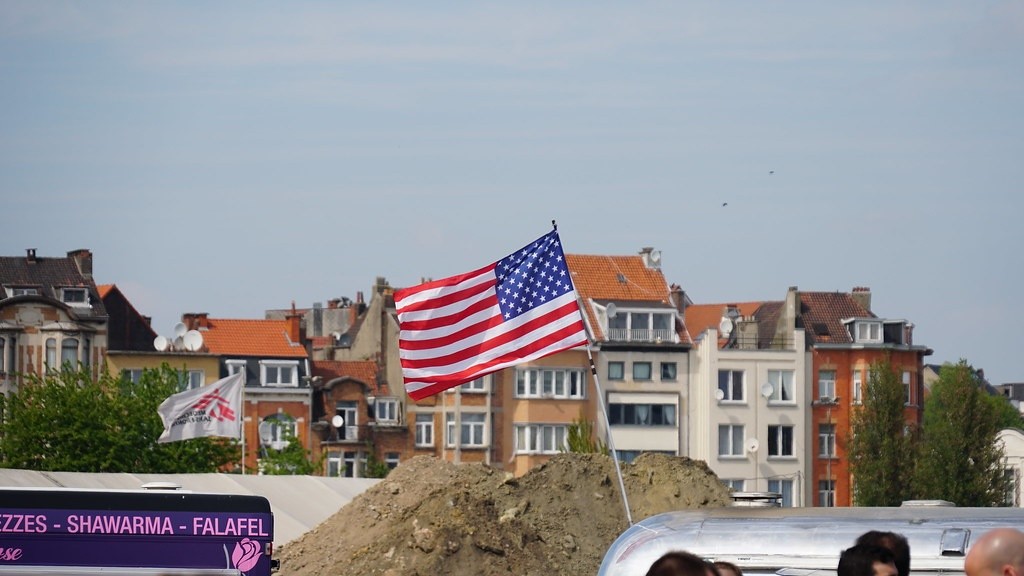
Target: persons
[641,527,1023,575]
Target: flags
[392,229,590,401]
[156,369,242,445]
